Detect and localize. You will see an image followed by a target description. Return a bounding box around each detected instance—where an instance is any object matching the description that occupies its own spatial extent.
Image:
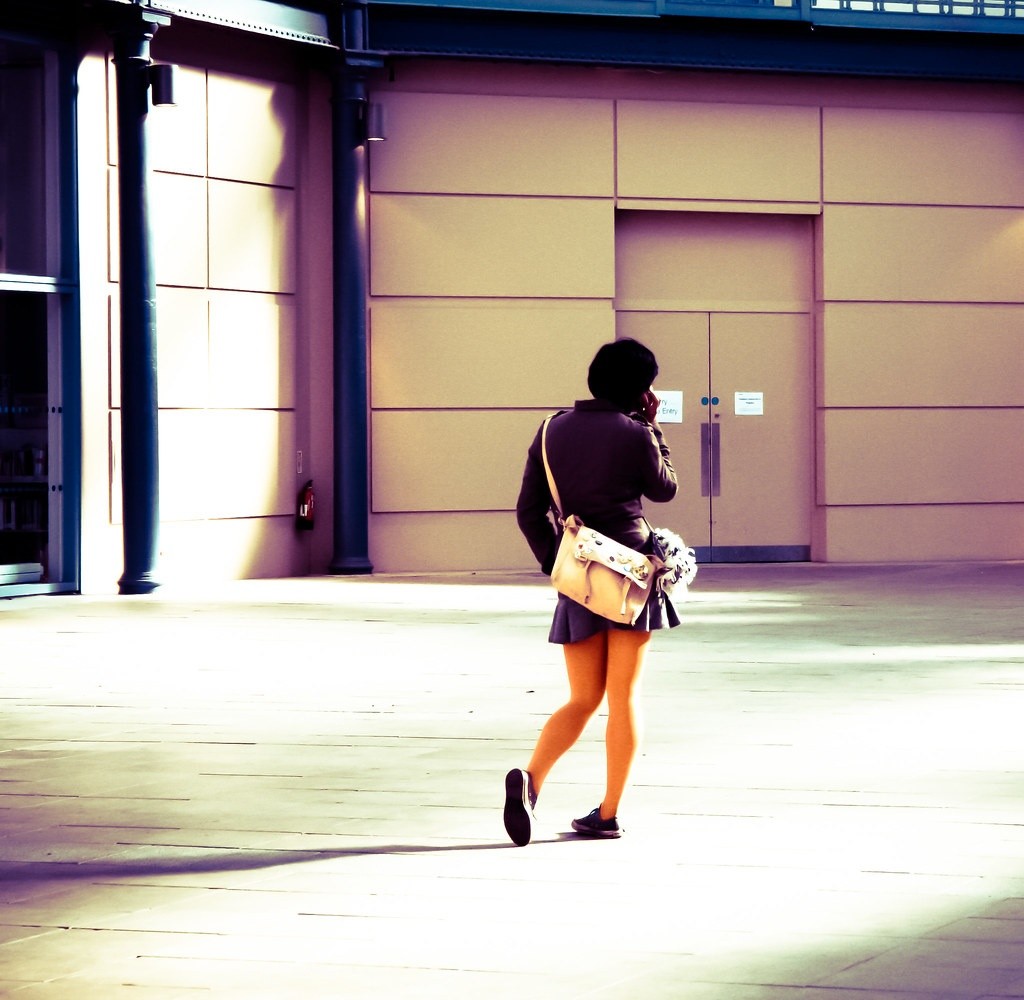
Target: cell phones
[640,392,653,408]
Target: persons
[502,335,677,847]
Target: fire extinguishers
[299,479,316,530]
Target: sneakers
[569,803,622,840]
[503,769,536,848]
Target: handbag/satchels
[547,518,663,626]
